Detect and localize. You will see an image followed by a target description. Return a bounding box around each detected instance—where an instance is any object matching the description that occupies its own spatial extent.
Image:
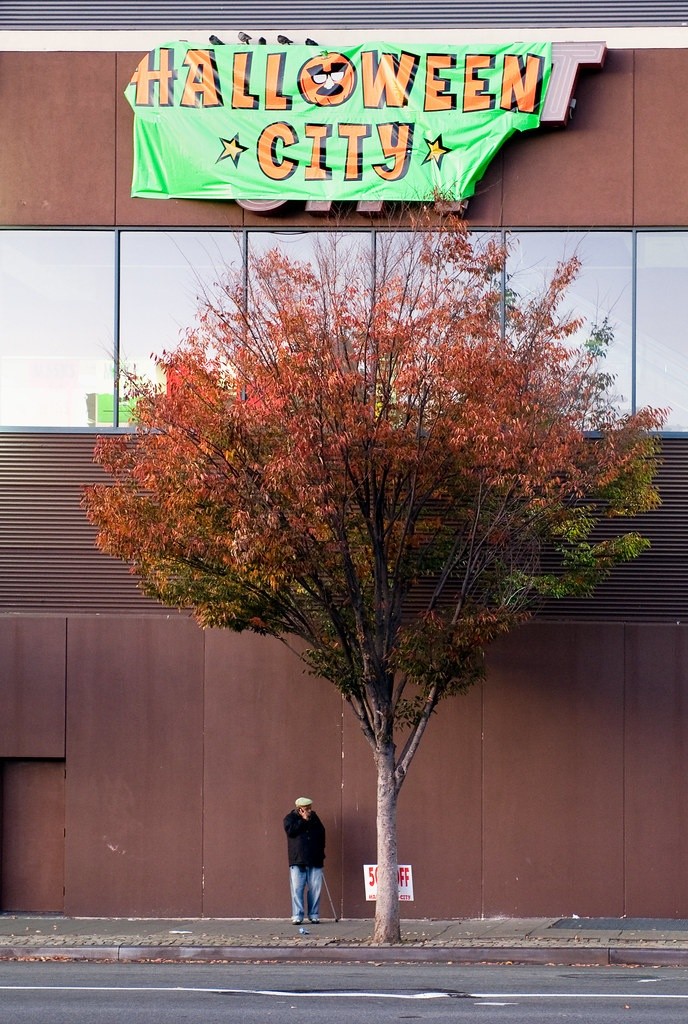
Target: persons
[282,797,326,926]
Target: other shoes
[311,919,320,923]
[292,920,301,925]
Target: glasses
[303,806,311,811]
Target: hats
[294,797,314,807]
